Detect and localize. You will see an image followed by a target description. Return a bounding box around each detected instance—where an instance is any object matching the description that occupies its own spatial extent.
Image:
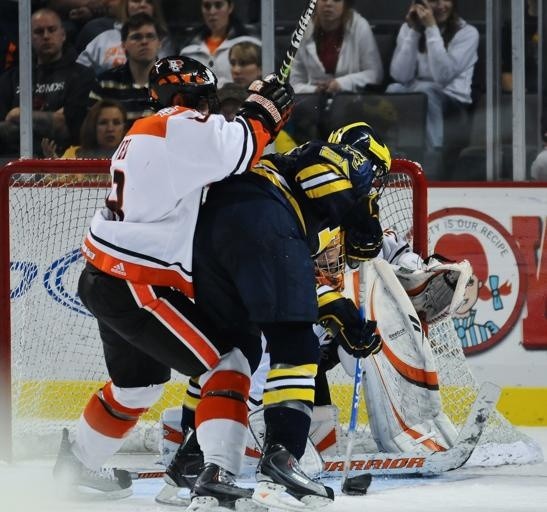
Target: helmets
[145,54,219,113]
[326,120,392,178]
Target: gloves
[315,295,384,359]
[240,72,296,139]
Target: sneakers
[51,426,133,493]
[254,436,337,511]
[163,424,269,512]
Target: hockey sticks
[341,259,371,495]
[129,381,502,480]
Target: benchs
[275,18,545,183]
